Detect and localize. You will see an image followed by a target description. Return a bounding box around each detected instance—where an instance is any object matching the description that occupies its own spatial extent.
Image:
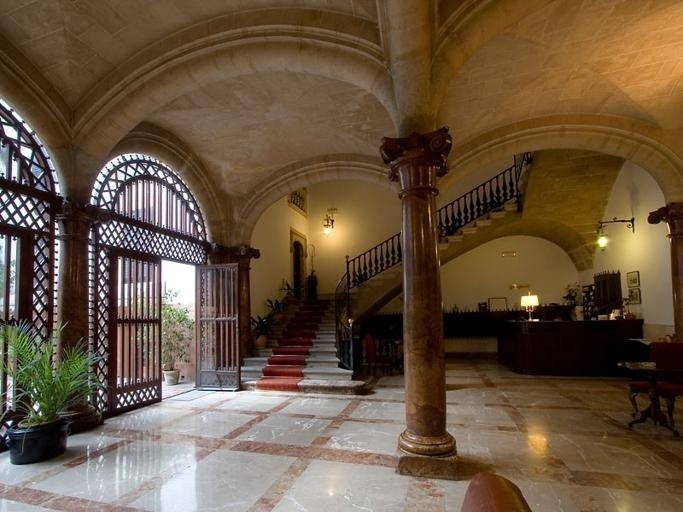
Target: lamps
[596,216,634,251]
[520,291,539,321]
[323,214,333,235]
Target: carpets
[255,298,330,391]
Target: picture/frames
[626,271,641,304]
[488,297,507,312]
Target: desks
[615,361,683,438]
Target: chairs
[627,341,683,425]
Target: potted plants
[0,315,111,466]
[249,299,285,350]
[131,288,194,385]
